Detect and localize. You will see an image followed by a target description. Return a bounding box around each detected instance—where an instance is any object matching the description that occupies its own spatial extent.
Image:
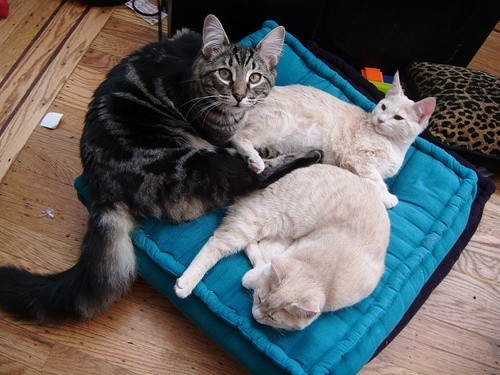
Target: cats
[231,69,437,210]
[173,165,391,331]
[0,13,324,326]
